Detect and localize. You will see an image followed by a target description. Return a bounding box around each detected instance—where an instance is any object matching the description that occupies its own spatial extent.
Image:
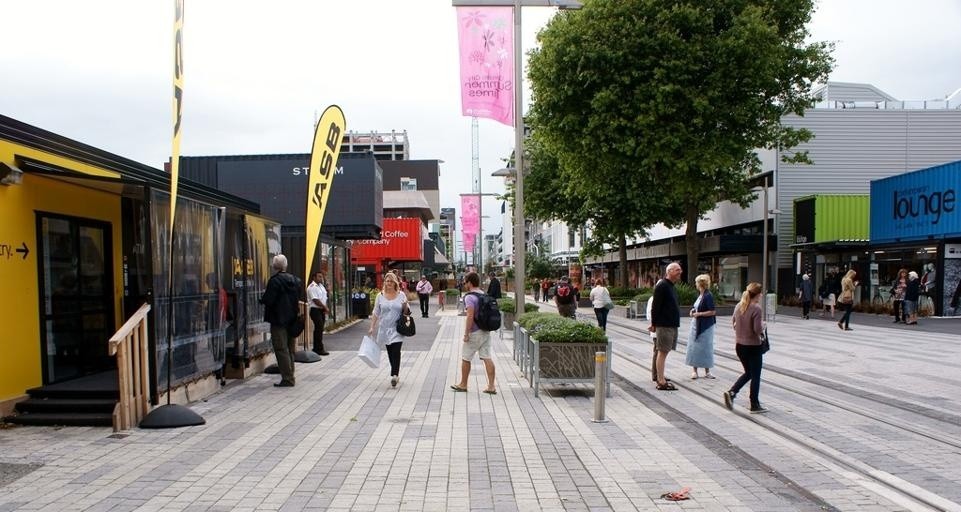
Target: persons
[449,272,498,393]
[723,281,769,414]
[307,271,330,355]
[261,254,305,388]
[368,269,435,387]
[532,276,580,319]
[590,278,611,332]
[645,262,685,389]
[798,267,858,329]
[921,264,932,316]
[204,272,228,386]
[950,279,961,316]
[890,268,921,325]
[925,262,938,316]
[686,275,718,379]
[486,272,503,300]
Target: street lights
[459,193,500,289]
[459,215,491,272]
[751,175,781,322]
[450,0,585,318]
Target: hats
[909,271,918,278]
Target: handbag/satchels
[600,289,615,310]
[357,335,380,369]
[759,327,770,355]
[394,305,416,338]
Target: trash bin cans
[355,293,370,318]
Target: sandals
[652,376,678,393]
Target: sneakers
[691,373,699,379]
[838,322,853,331]
[749,404,769,414]
[724,391,736,410]
[390,375,401,388]
[483,388,497,394]
[704,373,715,380]
[893,318,918,325]
[451,384,467,393]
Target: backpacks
[462,291,501,332]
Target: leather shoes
[273,376,295,387]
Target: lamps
[0,162,24,186]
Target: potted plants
[630,293,653,321]
[497,295,611,399]
[445,288,461,305]
